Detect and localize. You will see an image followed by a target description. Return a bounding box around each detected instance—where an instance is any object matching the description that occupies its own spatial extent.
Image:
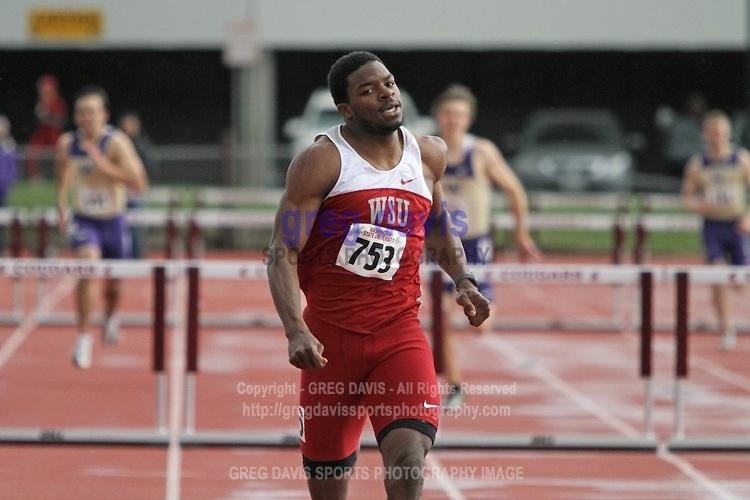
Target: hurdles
[0,185,750,452]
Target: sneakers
[67,317,118,369]
[442,387,463,414]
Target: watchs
[452,272,480,293]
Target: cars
[653,98,748,171]
[501,109,651,192]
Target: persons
[423,83,541,414]
[118,110,157,259]
[1,115,18,269]
[265,48,491,500]
[677,107,749,350]
[53,84,149,371]
[22,76,69,188]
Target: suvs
[281,87,439,160]
